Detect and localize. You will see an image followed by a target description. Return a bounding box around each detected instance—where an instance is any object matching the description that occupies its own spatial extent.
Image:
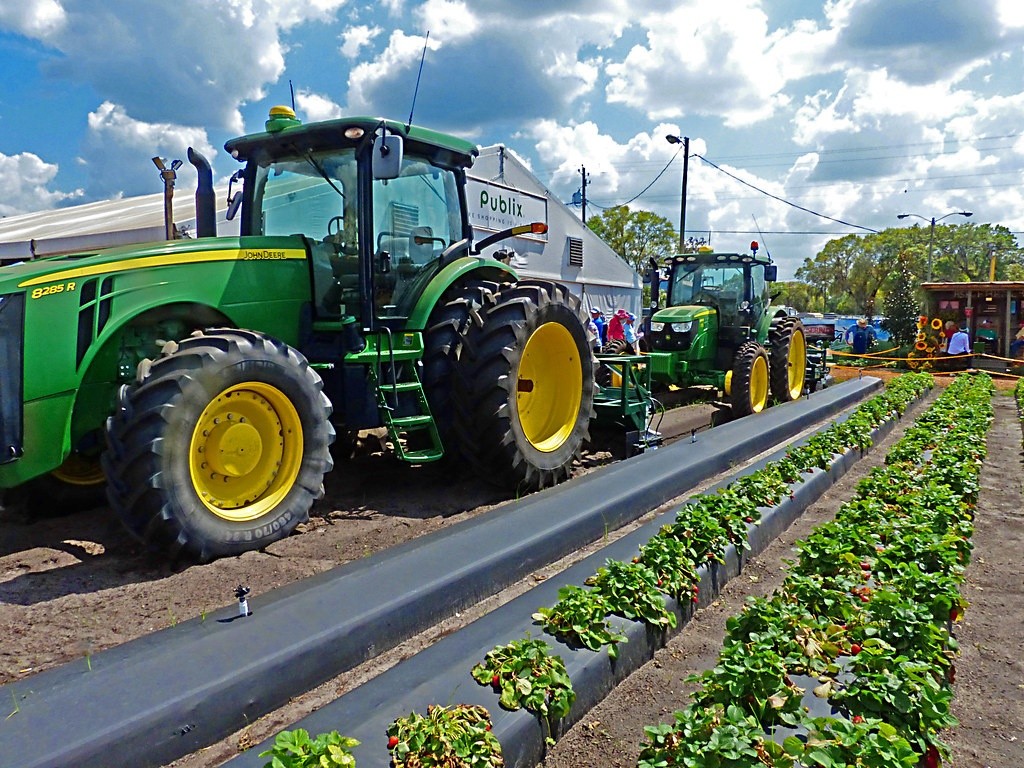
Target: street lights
[897,211,973,283]
[666,134,689,254]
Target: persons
[1006,327,1024,373]
[845,319,877,354]
[976,320,998,352]
[947,326,974,371]
[588,307,637,347]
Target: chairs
[390,226,434,271]
[718,291,737,316]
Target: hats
[590,306,600,314]
[959,326,970,333]
[614,309,631,319]
[627,313,637,321]
[856,318,868,327]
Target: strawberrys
[387,513,968,768]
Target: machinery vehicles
[601,241,826,418]
[0,96,652,556]
